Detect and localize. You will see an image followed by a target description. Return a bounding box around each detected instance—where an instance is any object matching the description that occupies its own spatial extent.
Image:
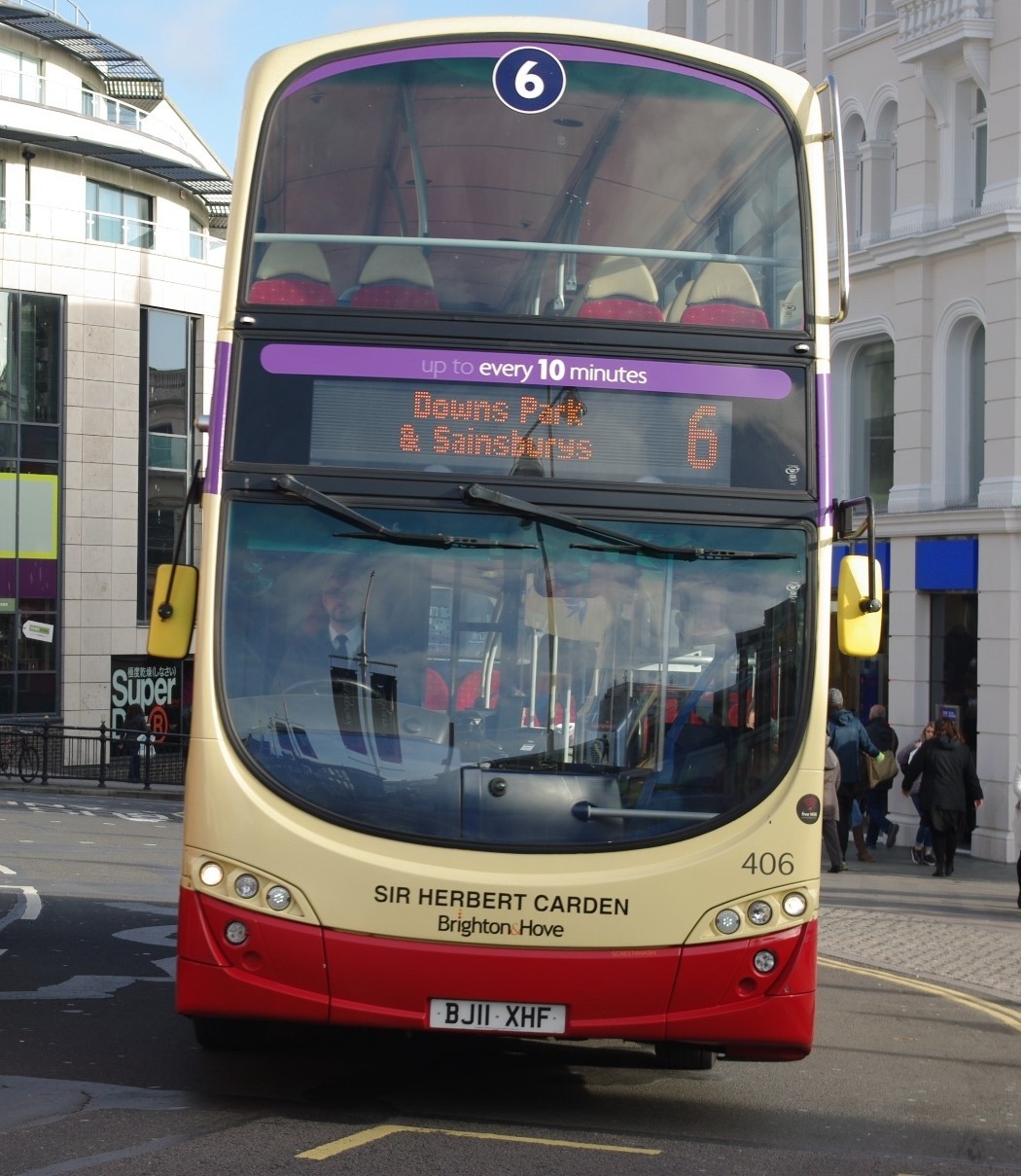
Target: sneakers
[910,846,924,864]
[922,853,936,866]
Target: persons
[901,717,983,877]
[1012,760,1021,910]
[897,722,936,866]
[120,704,150,780]
[851,799,878,863]
[827,689,885,871]
[272,570,386,696]
[859,705,899,848]
[823,734,843,873]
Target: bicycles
[0,729,44,783]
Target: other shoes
[826,868,841,873]
[865,841,876,849]
[841,862,849,871]
[933,870,944,877]
[945,867,954,876]
[886,824,899,849]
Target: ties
[335,634,349,672]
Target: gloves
[874,751,885,764]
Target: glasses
[324,588,362,597]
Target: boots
[853,823,876,862]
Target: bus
[147,15,885,1072]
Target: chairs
[243,240,440,313]
[573,256,769,328]
[423,663,755,757]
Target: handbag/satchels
[864,727,898,789]
[137,735,156,759]
[907,741,923,796]
